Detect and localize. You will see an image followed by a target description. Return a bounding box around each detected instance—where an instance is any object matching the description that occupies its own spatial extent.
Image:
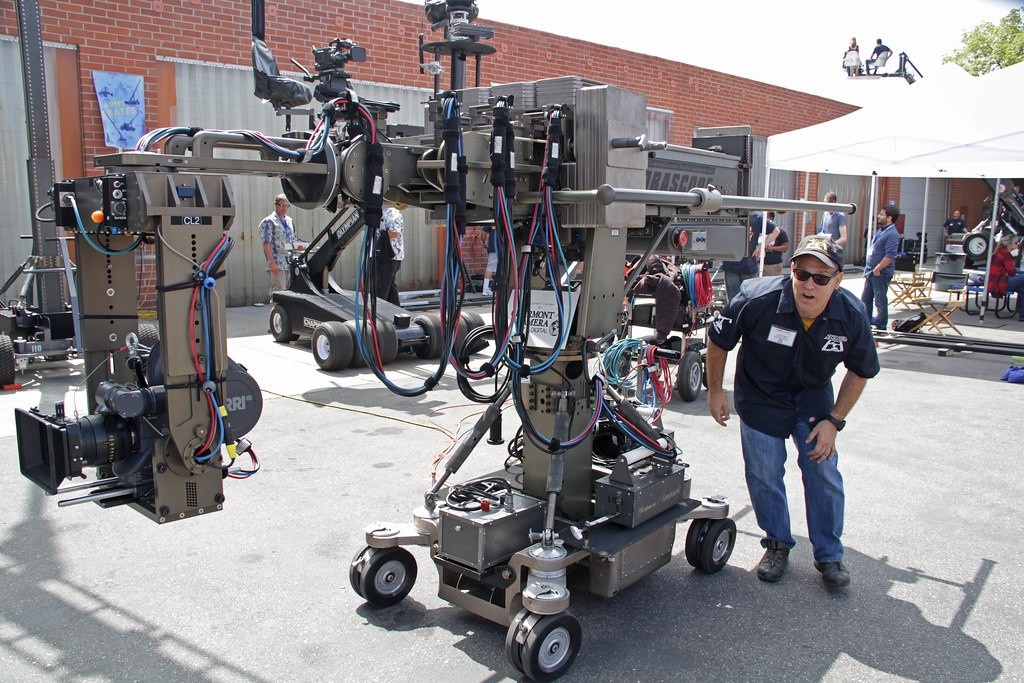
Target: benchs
[950,282,1014,319]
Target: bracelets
[1002,211,1006,214]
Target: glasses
[793,261,840,286]
[276,203,290,208]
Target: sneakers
[813,557,850,588]
[757,546,789,583]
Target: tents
[761,61,1024,325]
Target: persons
[865,38,893,76]
[370,201,404,308]
[842,37,861,77]
[758,211,789,276]
[717,213,780,306]
[707,234,879,588]
[942,208,969,237]
[861,205,900,330]
[987,233,1024,322]
[259,194,305,335]
[481,225,498,296]
[984,186,1024,225]
[813,191,848,247]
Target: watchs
[824,414,846,432]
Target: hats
[788,233,843,272]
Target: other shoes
[482,288,493,296]
[1019,317,1024,321]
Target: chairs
[886,271,968,336]
[843,50,888,77]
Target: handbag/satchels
[892,313,927,332]
